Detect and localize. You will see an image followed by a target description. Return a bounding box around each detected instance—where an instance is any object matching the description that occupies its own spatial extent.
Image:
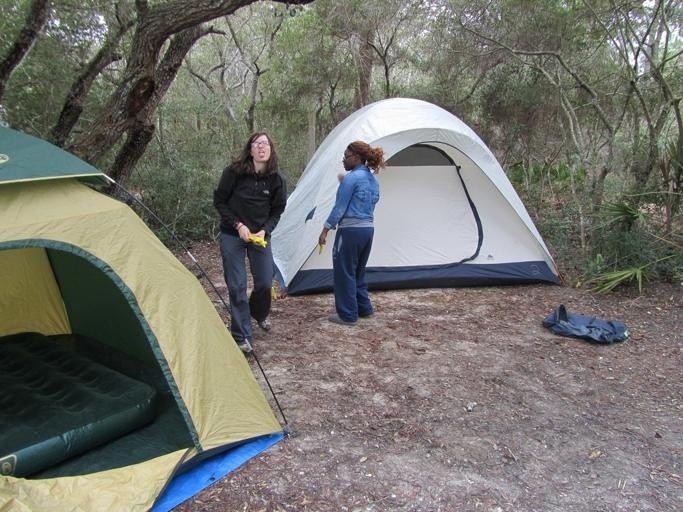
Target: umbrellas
[0,120,288,510]
[267,95,567,299]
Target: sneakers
[257,316,273,331]
[327,312,375,326]
[237,338,254,354]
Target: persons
[212,132,288,353]
[316,141,386,326]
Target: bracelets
[237,223,245,231]
